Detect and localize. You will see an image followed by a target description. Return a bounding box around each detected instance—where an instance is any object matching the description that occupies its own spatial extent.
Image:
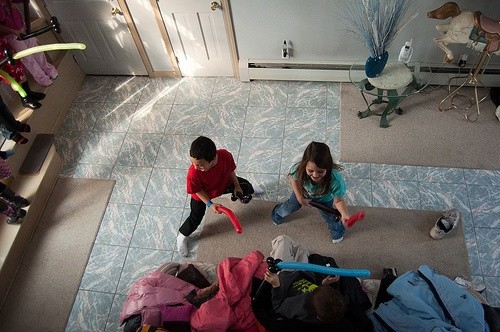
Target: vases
[365,51,389,79]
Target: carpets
[0,176,121,332]
[339,81,500,171]
[193,197,472,280]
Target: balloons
[253,256,371,296]
[0,16,86,97]
[309,200,341,222]
[344,210,368,228]
[231,191,252,203]
[217,206,243,234]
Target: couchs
[123,260,383,332]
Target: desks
[348,50,432,129]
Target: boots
[0,198,26,224]
[22,81,47,100]
[0,186,30,207]
[19,91,42,109]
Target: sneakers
[430,209,460,240]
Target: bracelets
[206,201,213,207]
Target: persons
[0,182,30,224]
[0,96,31,145]
[272,141,352,244]
[0,0,58,109]
[176,136,264,258]
[265,271,366,326]
